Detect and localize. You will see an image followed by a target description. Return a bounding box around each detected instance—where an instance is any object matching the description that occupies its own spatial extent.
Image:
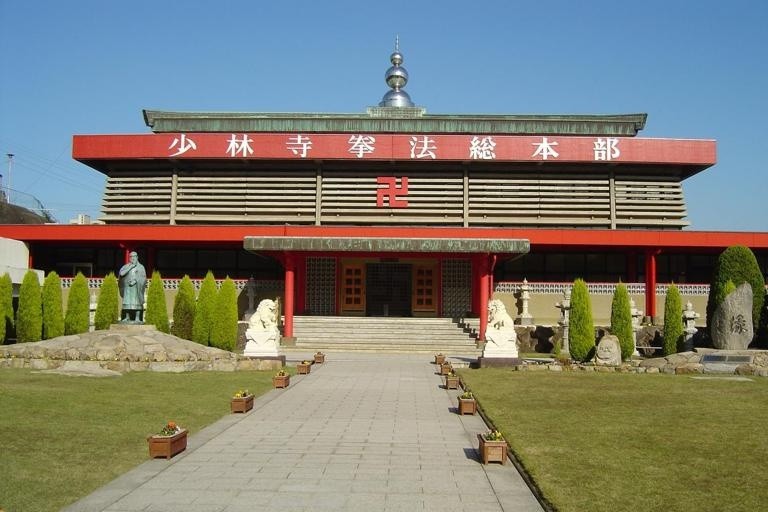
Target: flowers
[462,390,472,399]
[486,430,503,441]
[234,390,249,398]
[157,421,179,436]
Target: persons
[117,248,149,321]
